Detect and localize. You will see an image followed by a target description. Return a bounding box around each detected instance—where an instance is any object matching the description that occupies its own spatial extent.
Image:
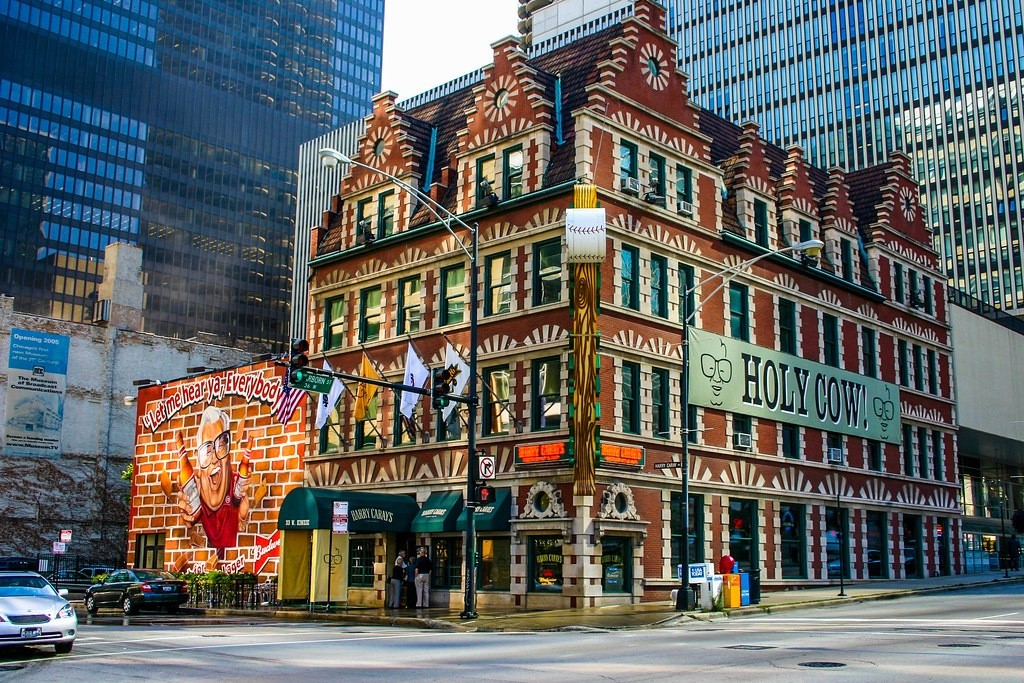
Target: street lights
[677,238,824,605]
[317,147,480,620]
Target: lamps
[252,353,283,363]
[123,395,139,406]
[356,234,373,244]
[804,256,819,268]
[133,378,167,386]
[477,193,502,209]
[186,366,218,373]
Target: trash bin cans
[739,568,761,604]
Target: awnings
[276,487,421,614]
[411,490,463,532]
[456,487,511,531]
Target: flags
[353,351,381,422]
[400,342,429,420]
[276,367,306,427]
[442,343,470,421]
[314,359,345,429]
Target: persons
[414,549,433,609]
[406,557,416,583]
[394,550,407,568]
[387,558,405,608]
[1007,534,1022,571]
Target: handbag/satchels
[386,575,392,584]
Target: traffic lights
[435,368,450,408]
[478,487,492,504]
[291,339,310,384]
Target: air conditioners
[620,177,640,194]
[677,201,692,216]
[827,448,842,463]
[732,432,751,449]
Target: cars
[0,571,78,654]
[0,556,118,586]
[85,568,190,615]
[828,547,920,576]
